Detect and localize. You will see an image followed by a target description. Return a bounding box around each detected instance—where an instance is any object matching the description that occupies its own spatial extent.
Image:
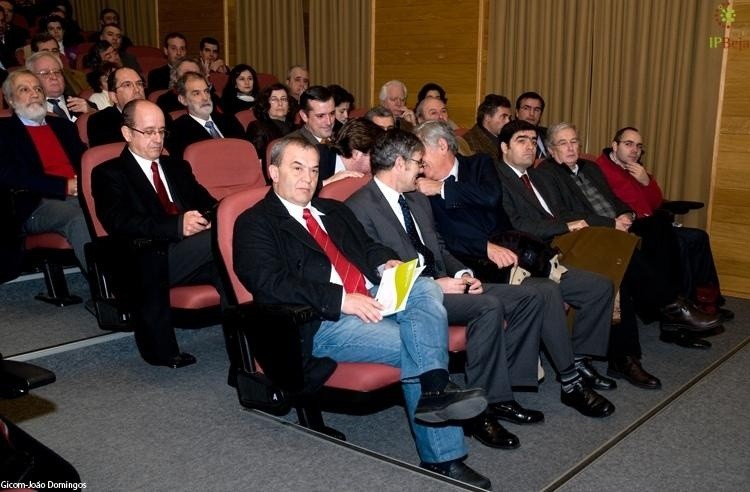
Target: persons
[92,99,224,295]
[415,120,617,417]
[596,127,735,322]
[232,135,491,490]
[343,129,544,450]
[0,68,92,276]
[0,0,551,191]
[535,120,713,350]
[496,119,723,391]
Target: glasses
[34,69,61,79]
[130,127,166,137]
[116,81,147,89]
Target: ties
[520,175,536,205]
[398,194,435,271]
[302,208,373,299]
[150,160,178,218]
[47,99,68,120]
[206,121,223,140]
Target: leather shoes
[153,351,197,368]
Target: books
[374,258,427,317]
